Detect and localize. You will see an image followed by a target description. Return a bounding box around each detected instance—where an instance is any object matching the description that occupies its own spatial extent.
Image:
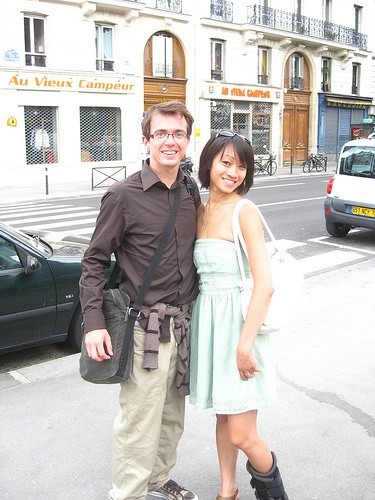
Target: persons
[192,128,289,500]
[77,100,200,500]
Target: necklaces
[203,196,215,230]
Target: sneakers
[149,481,198,500]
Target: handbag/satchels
[79,287,135,384]
[233,199,305,333]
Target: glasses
[149,131,188,143]
[216,129,250,144]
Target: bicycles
[253,150,278,177]
[302,149,326,173]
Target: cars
[0,220,128,355]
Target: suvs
[324,132,375,238]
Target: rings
[244,373,250,377]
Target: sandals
[217,488,240,500]
